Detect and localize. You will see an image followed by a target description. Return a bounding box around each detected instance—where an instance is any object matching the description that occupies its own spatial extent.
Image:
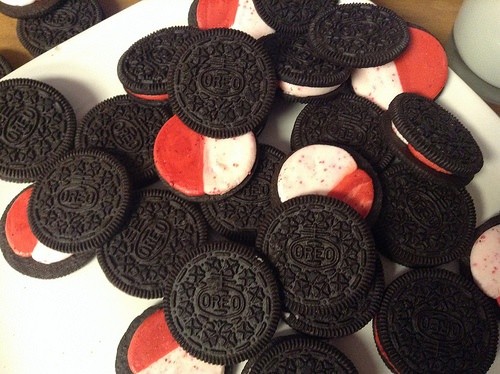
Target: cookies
[0,79,77,183]
[0,148,133,280]
[74,95,172,189]
[0,56,12,79]
[1,0,107,55]
[114,0,499,374]
